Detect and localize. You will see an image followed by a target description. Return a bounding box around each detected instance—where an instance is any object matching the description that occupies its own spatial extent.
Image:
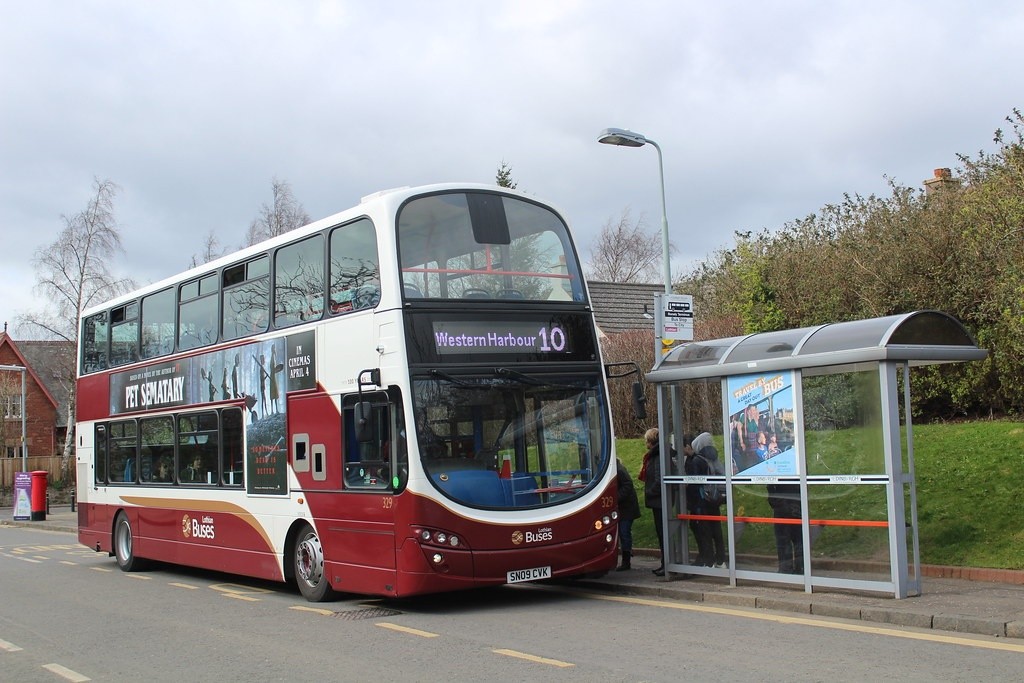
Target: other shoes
[652,568,664,576]
[692,556,727,568]
[793,568,805,575]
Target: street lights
[600,128,690,577]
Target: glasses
[160,465,168,469]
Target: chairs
[109,457,188,483]
[84,313,288,374]
[403,283,423,298]
[350,287,379,310]
[495,289,525,300]
[463,288,492,299]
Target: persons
[615,456,639,571]
[184,456,208,483]
[730,404,780,461]
[155,460,173,483]
[769,483,804,576]
[640,428,732,575]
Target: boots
[615,548,632,572]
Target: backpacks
[697,454,730,503]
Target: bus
[75,184,648,605]
